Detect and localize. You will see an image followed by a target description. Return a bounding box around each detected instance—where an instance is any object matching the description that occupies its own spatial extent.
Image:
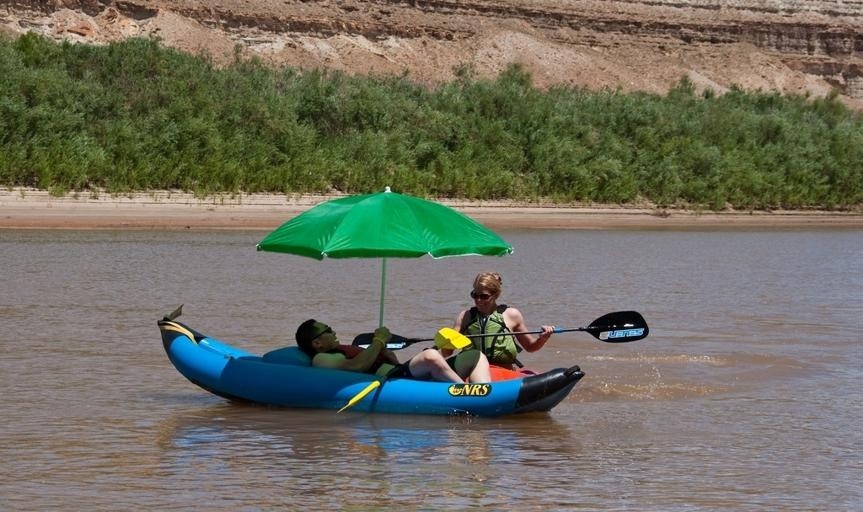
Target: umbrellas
[255,186,514,329]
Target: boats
[157,303,584,418]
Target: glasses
[471,290,491,300]
[312,327,332,340]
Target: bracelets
[372,337,386,349]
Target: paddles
[352,311,648,350]
[336,327,472,414]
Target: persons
[441,272,555,370]
[295,319,491,383]
